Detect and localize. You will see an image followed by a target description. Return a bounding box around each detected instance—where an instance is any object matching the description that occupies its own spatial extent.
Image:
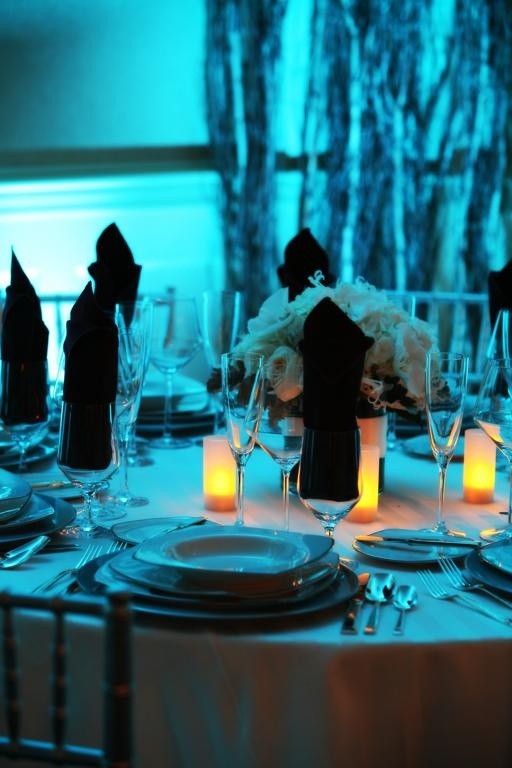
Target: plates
[351,525,478,567]
[0,466,76,545]
[465,540,511,592]
[75,515,344,624]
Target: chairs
[1,584,134,768]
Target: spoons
[3,536,51,568]
[363,572,393,633]
[389,582,416,633]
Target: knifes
[344,571,369,633]
[353,535,482,547]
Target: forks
[63,540,130,595]
[29,543,103,595]
[438,557,510,609]
[416,568,510,626]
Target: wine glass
[3,291,512,510]
[58,399,118,539]
[252,364,309,534]
[220,350,267,530]
[423,350,468,538]
